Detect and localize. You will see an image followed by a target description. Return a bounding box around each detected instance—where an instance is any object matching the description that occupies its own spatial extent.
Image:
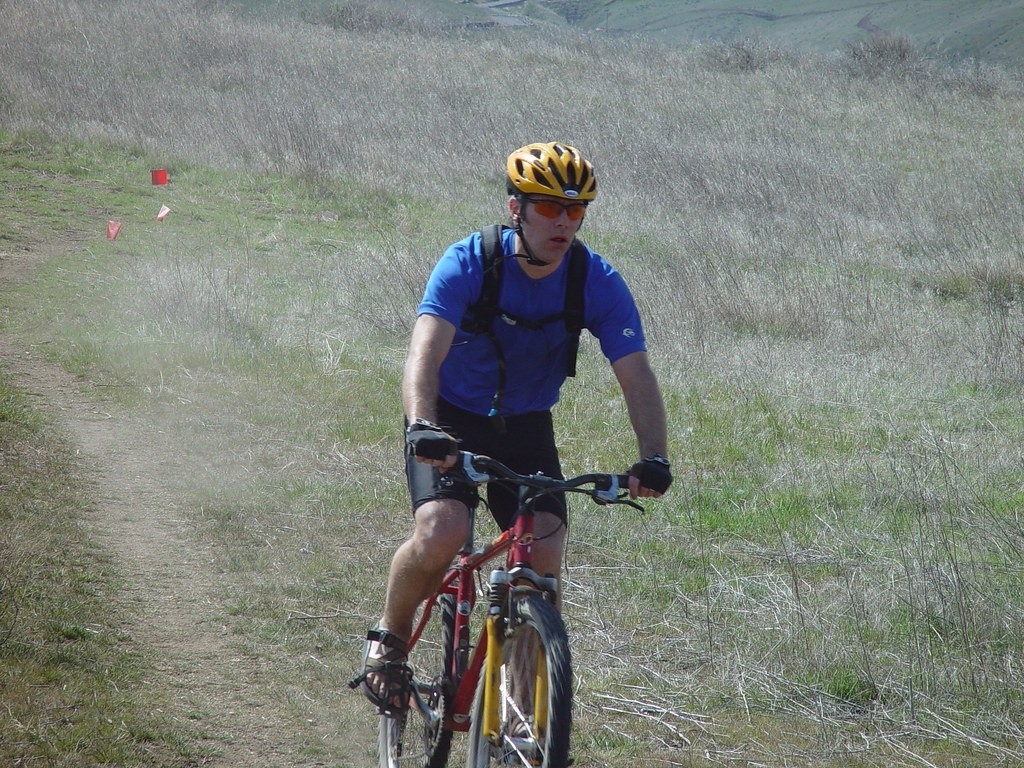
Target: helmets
[506,142,599,202]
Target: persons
[360,142,672,768]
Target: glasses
[525,198,586,220]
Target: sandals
[503,713,535,741]
[359,622,411,712]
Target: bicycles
[377,451,645,768]
